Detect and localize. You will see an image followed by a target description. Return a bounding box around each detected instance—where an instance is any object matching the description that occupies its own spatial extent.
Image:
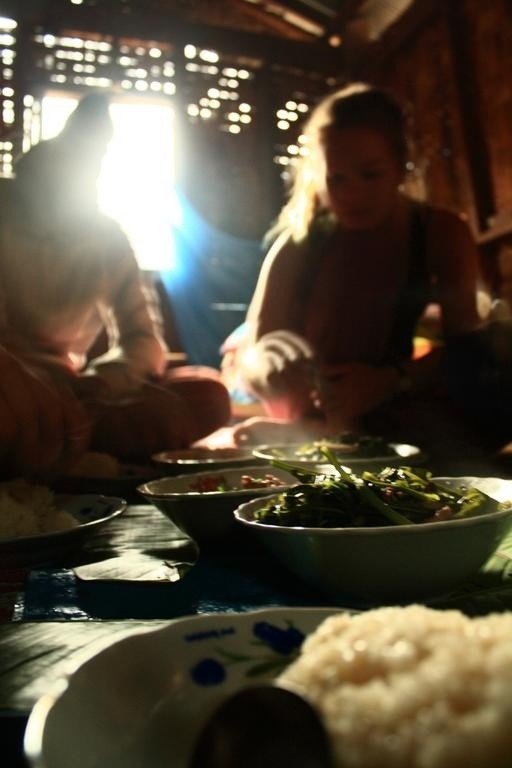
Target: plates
[0,488,127,552]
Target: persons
[220,87,512,454]
[0,140,229,485]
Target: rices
[269,606,512,768]
[2,485,81,541]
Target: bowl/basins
[21,607,362,762]
[151,446,263,477]
[233,474,511,598]
[135,463,352,558]
[252,441,421,473]
